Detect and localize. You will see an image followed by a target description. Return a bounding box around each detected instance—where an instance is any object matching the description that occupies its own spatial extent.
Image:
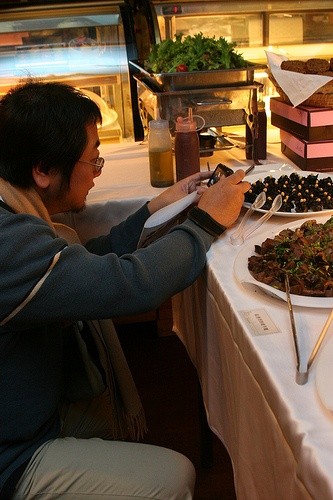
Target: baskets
[268,76,333,107]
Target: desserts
[243,173,333,211]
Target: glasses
[78,157,104,173]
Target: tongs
[284,273,333,385]
[230,191,282,244]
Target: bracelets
[189,207,224,236]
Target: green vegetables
[259,215,333,290]
[145,31,246,74]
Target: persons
[0,81,251,500]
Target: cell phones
[207,163,233,187]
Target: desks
[151,216,333,500]
[51,140,302,244]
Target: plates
[242,170,333,218]
[234,217,333,308]
[96,109,118,128]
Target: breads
[280,57,333,79]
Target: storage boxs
[271,96,333,140]
[280,129,333,172]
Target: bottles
[246,88,267,161]
[175,106,206,181]
[148,120,175,187]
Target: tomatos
[178,65,187,72]
